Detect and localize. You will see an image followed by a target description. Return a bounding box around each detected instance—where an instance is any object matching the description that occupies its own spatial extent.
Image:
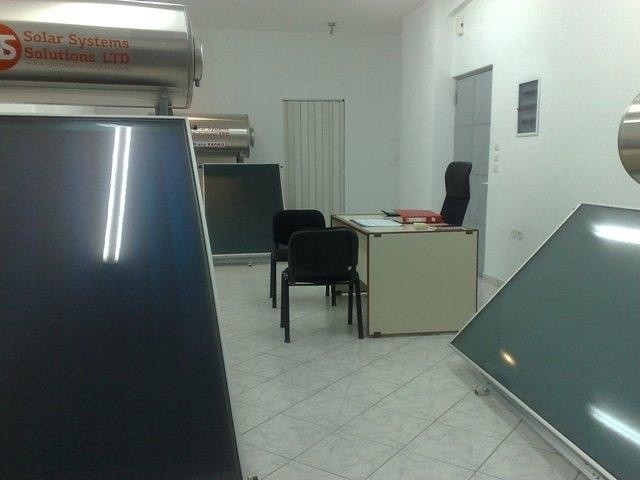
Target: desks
[331,213,479,336]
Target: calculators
[381,208,400,216]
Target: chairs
[439,162,472,225]
[270,209,326,308]
[280,229,363,341]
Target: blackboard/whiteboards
[447,201,640,480]
